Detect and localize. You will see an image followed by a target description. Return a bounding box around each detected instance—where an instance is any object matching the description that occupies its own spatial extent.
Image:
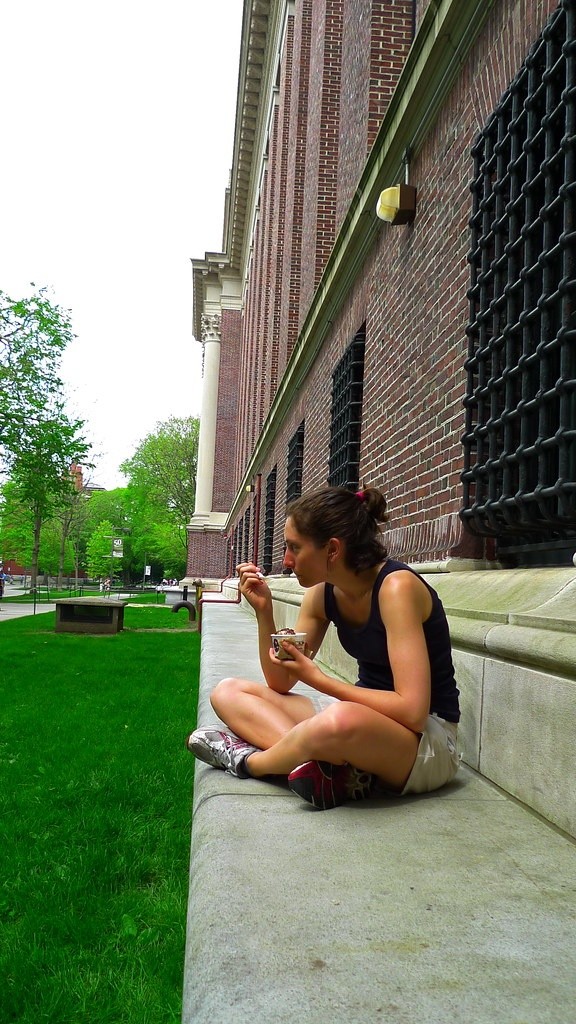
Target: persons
[20,574,24,583]
[187,486,462,811]
[0,568,6,611]
[163,578,178,586]
[9,574,14,583]
[100,580,110,592]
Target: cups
[270,633,306,661]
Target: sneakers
[287,760,377,810]
[187,725,264,779]
